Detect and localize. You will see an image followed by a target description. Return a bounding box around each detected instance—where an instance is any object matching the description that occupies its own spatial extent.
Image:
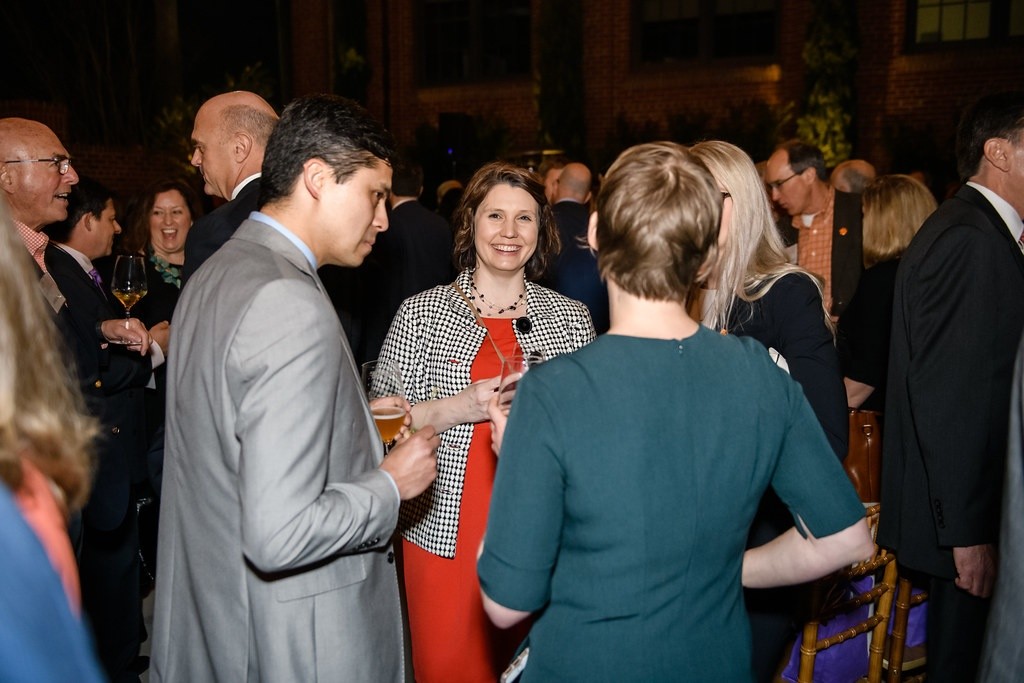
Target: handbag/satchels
[844,408,880,502]
[781,570,927,683]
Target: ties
[90,267,107,301]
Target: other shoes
[137,656,150,674]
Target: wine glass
[110,255,149,344]
[360,359,407,457]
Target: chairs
[778,503,930,683]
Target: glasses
[5,158,73,174]
[766,167,809,192]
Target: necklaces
[472,278,523,316]
[146,247,182,289]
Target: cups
[496,354,544,416]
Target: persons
[0,90,1024,683]
